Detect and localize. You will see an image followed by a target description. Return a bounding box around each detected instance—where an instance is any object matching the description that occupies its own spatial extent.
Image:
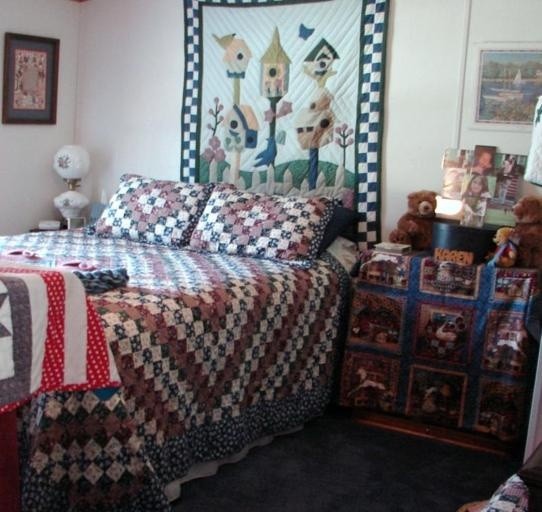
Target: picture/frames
[3,31,62,126]
[473,40,542,133]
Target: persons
[471,151,493,175]
[502,154,517,177]
[463,176,493,199]
[459,198,474,226]
[475,197,488,228]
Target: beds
[2,174,366,508]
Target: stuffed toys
[512,194,542,267]
[489,226,518,268]
[389,191,437,249]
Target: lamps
[53,143,91,223]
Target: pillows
[94,174,341,269]
